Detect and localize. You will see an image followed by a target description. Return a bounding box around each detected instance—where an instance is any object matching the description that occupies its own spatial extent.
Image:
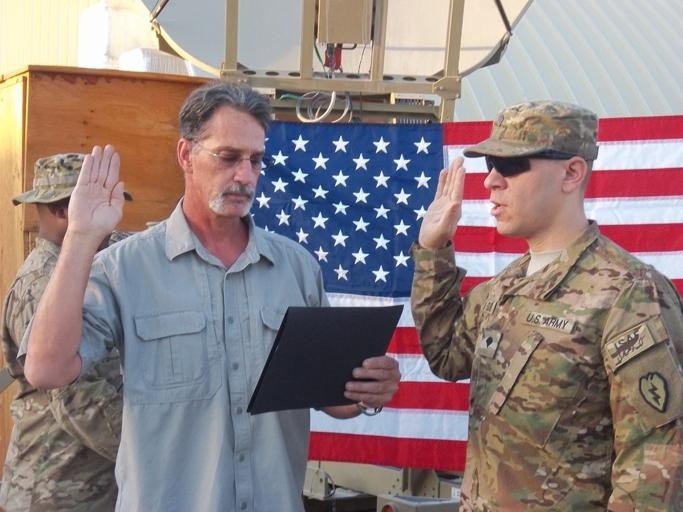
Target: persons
[14,84,402,511]
[0,152,138,512]
[410,101,683,512]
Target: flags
[249,115,683,473]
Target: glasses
[193,139,262,171]
[486,155,574,178]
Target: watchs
[357,402,384,416]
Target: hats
[462,99,599,160]
[11,152,134,206]
